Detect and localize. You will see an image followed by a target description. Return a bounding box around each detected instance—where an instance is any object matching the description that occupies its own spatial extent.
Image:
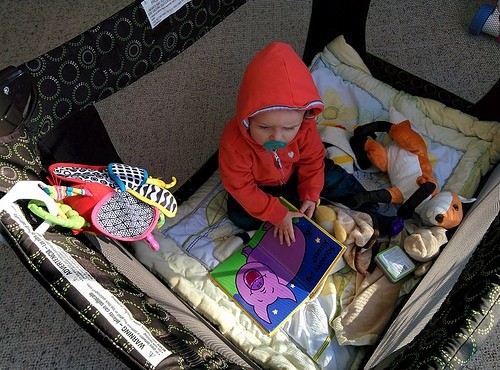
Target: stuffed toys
[404,226,449,277]
[353,121,477,230]
[310,205,347,241]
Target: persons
[219,42,393,229]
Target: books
[374,244,416,284]
[208,196,349,338]
